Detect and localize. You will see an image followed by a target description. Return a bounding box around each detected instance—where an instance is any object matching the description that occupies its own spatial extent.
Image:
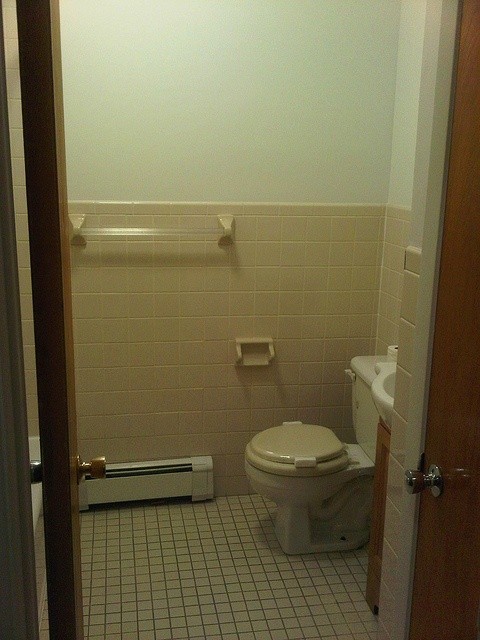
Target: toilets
[245,351,386,555]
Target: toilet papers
[387,345,397,359]
[244,352,269,367]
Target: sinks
[370,360,399,430]
